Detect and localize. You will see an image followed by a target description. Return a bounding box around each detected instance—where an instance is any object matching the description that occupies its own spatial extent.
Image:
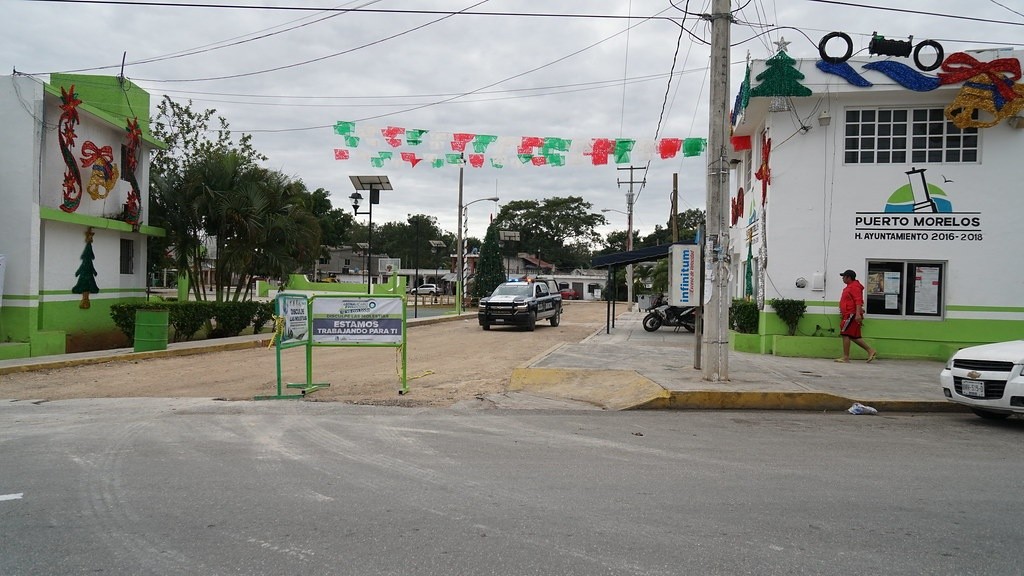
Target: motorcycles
[642,292,704,333]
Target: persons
[833,269,876,363]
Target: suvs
[477,277,563,332]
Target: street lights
[356,242,374,284]
[601,190,634,312]
[408,214,419,318]
[430,240,447,303]
[348,175,394,294]
[456,153,499,315]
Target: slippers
[834,357,849,363]
[866,351,877,363]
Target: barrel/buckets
[133,309,169,352]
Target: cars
[252,276,270,290]
[411,284,444,296]
[406,282,410,294]
[939,340,1024,421]
[559,288,577,300]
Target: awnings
[591,239,696,334]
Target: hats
[840,270,856,278]
